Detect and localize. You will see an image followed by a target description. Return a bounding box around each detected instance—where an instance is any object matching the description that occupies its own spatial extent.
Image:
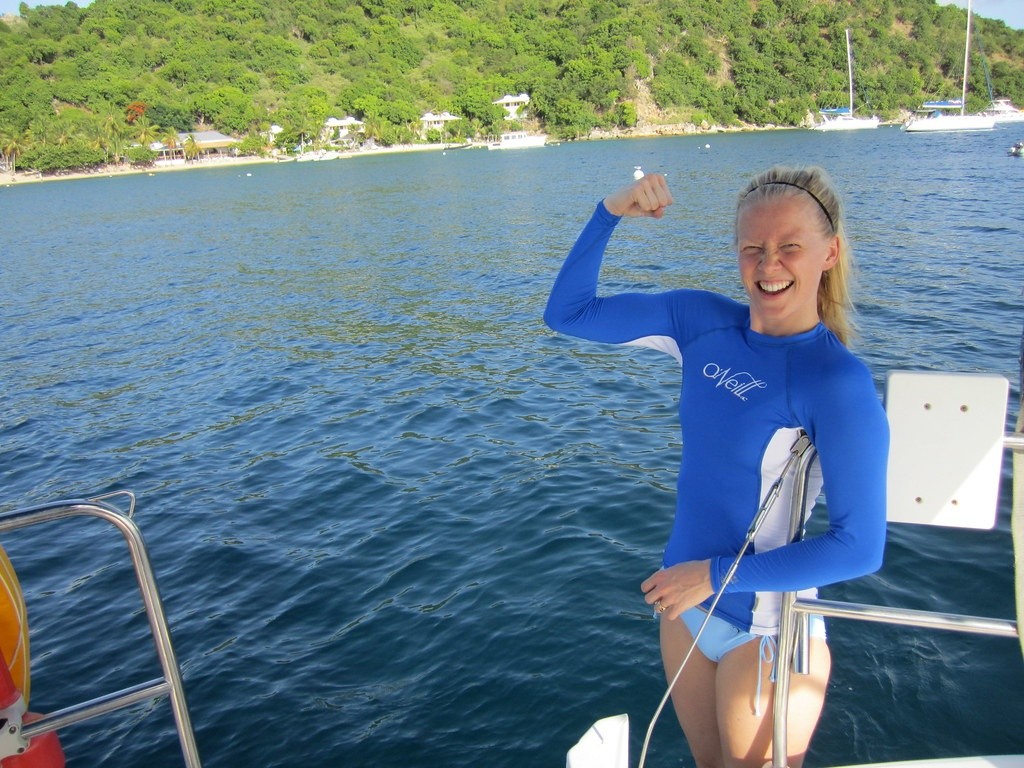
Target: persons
[545,166,888,768]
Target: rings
[657,601,666,612]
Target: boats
[818,107,852,116]
[1008,141,1023,156]
[977,98,1024,123]
[923,99,964,111]
[444,142,472,151]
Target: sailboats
[812,28,881,133]
[897,0,996,133]
